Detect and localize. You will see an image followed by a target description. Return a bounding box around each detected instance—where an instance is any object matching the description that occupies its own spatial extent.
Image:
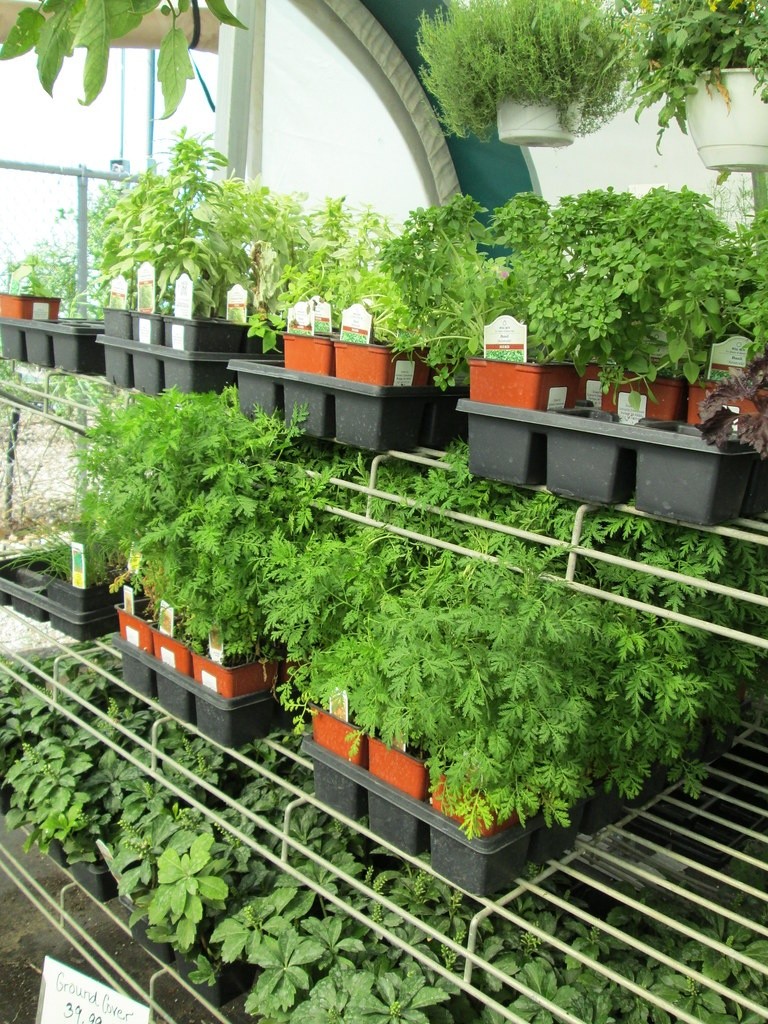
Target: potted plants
[414,0,641,146]
[0,130,768,1006]
[610,0,768,182]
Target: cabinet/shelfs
[3,368,768,1024]
